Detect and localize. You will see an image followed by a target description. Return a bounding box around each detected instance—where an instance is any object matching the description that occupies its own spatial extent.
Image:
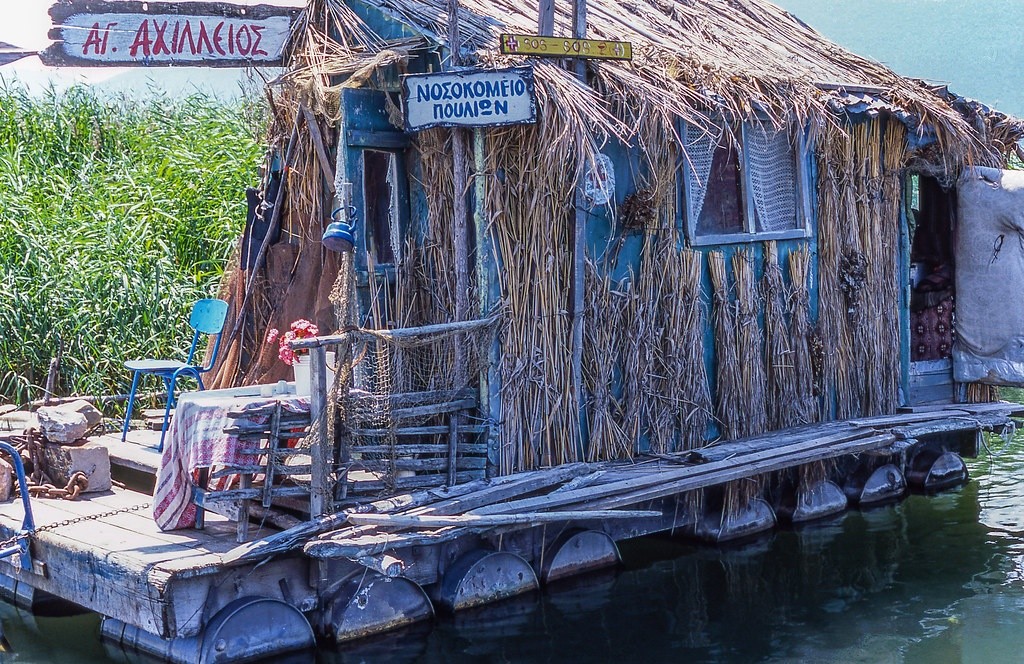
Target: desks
[152,381,311,544]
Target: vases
[292,351,336,396]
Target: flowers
[267,319,318,367]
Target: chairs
[121,299,229,453]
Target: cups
[260,384,273,397]
[276,380,288,393]
